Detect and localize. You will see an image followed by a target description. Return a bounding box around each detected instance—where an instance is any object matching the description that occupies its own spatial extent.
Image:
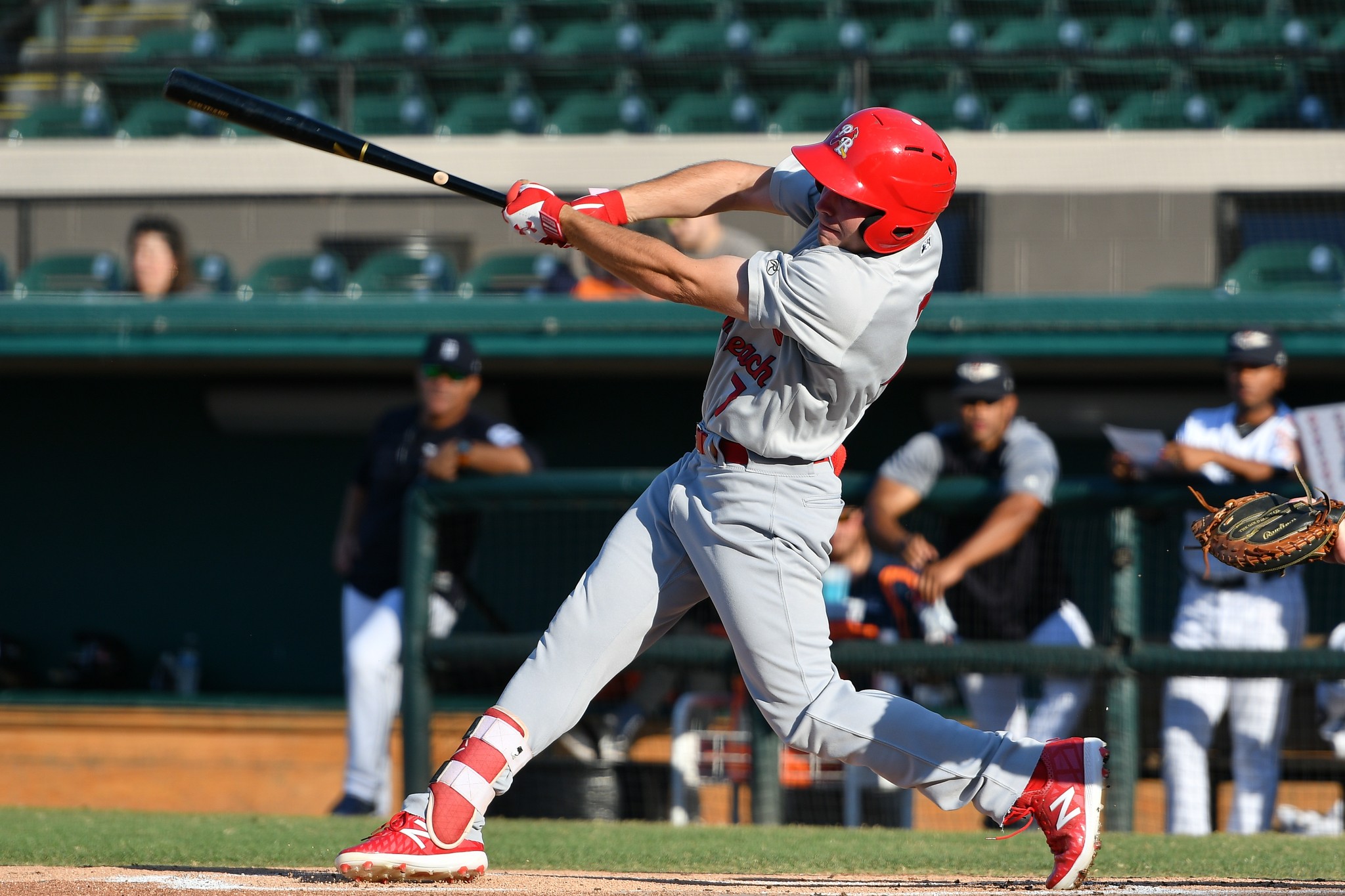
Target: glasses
[421,364,469,381]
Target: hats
[951,351,1015,402]
[417,330,480,373]
[1223,329,1288,368]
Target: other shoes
[334,795,376,816]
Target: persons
[333,106,1108,894]
[1187,464,1345,581]
[1109,329,1306,836]
[832,360,1093,831]
[328,333,531,815]
[121,213,215,294]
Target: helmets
[791,106,957,254]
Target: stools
[673,631,918,826]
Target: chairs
[1223,241,1345,288]
[0,251,577,295]
[0,0,1345,138]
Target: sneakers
[1004,735,1110,891]
[334,811,488,884]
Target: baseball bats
[161,63,510,211]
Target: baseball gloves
[1180,460,1345,582]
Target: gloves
[502,179,629,248]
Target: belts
[1198,565,1285,591]
[695,423,830,467]
